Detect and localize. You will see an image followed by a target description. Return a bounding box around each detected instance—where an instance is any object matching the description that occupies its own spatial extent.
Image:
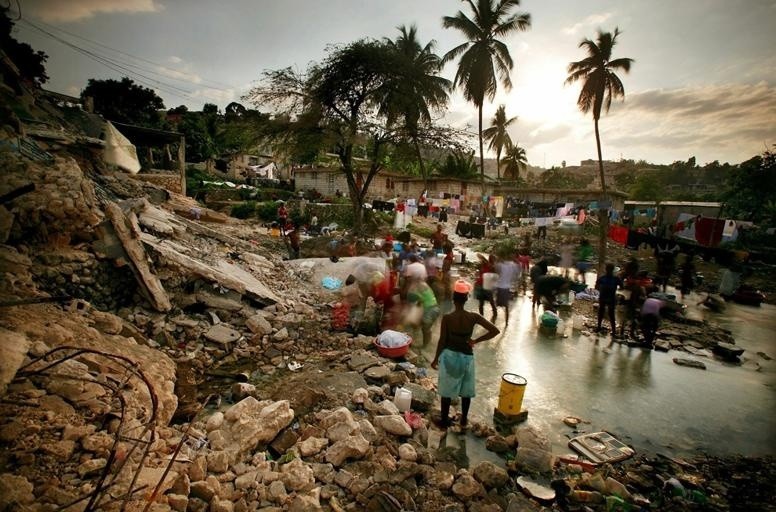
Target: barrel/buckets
[498,372,527,416]
[573,312,584,330]
[394,387,412,414]
[453,253,462,264]
[584,271,598,289]
[311,216,318,226]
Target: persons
[429,291,501,433]
[276,186,698,350]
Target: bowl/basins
[374,333,413,358]
